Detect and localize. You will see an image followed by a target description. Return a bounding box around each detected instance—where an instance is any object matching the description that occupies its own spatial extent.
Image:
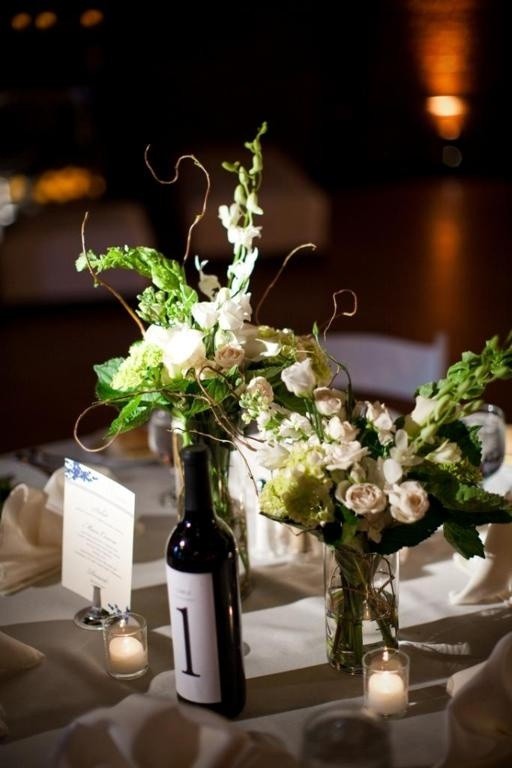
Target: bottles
[163,441,249,722]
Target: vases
[321,546,400,676]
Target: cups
[101,611,151,682]
[295,700,395,768]
[360,647,413,721]
[458,402,507,479]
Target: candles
[362,649,412,712]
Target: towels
[443,629,512,768]
[0,462,151,681]
